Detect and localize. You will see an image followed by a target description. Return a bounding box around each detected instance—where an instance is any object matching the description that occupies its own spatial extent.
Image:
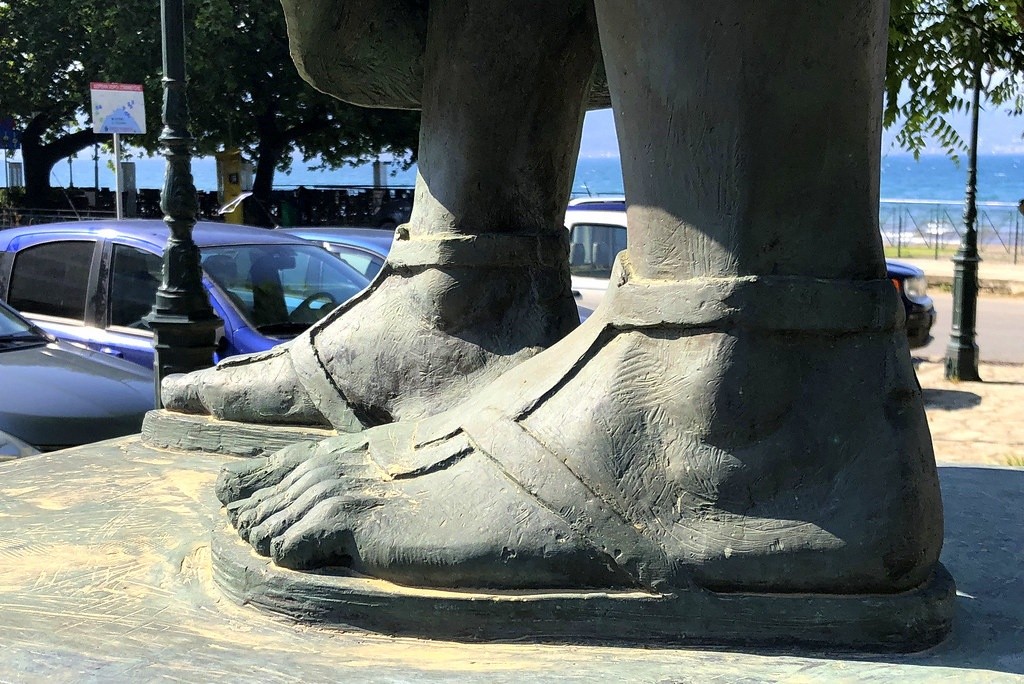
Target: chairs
[116,249,162,323]
[200,255,249,329]
[570,241,586,272]
[593,241,609,270]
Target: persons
[202,255,253,325]
[141,0,957,655]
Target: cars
[0,214,373,454]
[214,222,396,316]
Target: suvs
[563,196,939,350]
